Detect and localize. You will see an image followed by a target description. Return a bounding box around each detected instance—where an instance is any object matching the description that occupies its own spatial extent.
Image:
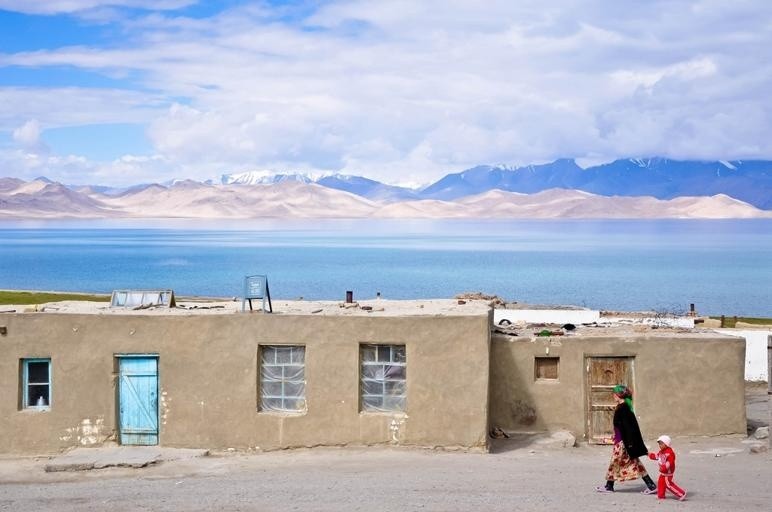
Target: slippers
[656,496,664,501]
[678,491,687,502]
[594,485,614,493]
[640,487,658,494]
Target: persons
[646,434,688,502]
[592,383,657,494]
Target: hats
[656,435,672,447]
